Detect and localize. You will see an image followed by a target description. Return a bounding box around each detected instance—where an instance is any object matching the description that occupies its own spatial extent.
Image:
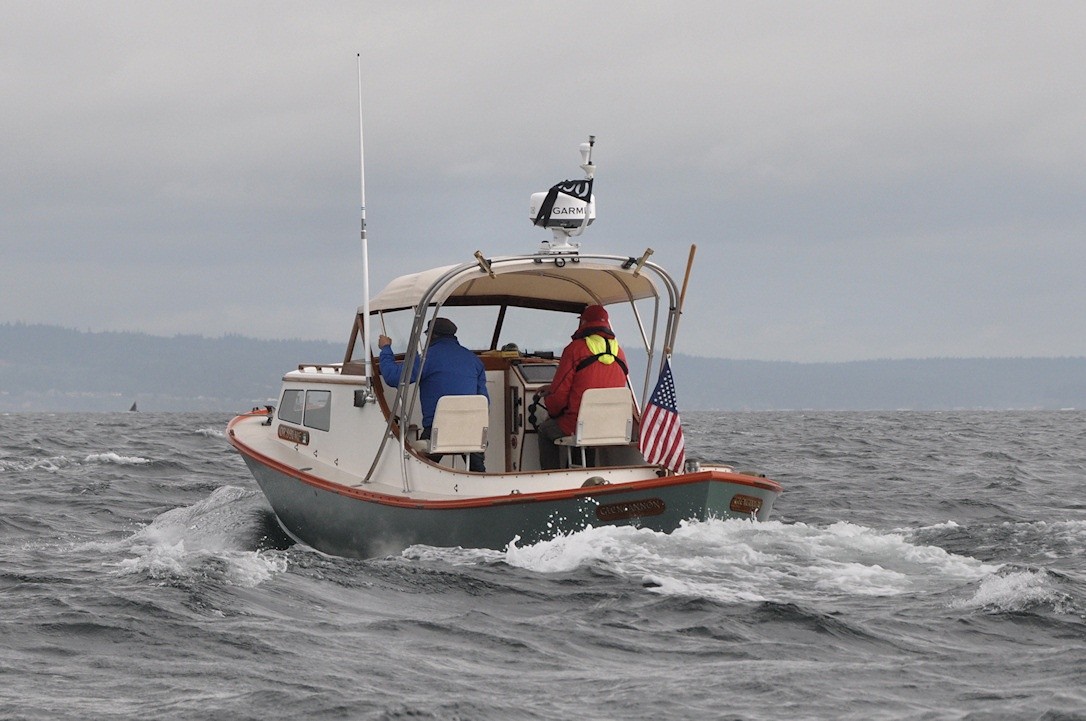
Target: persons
[378,318,489,472]
[537,305,629,469]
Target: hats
[578,304,610,323]
[424,318,457,335]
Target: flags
[637,357,686,475]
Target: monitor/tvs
[519,362,560,383]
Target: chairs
[554,388,634,468]
[414,394,490,472]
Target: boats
[226,49,784,562]
